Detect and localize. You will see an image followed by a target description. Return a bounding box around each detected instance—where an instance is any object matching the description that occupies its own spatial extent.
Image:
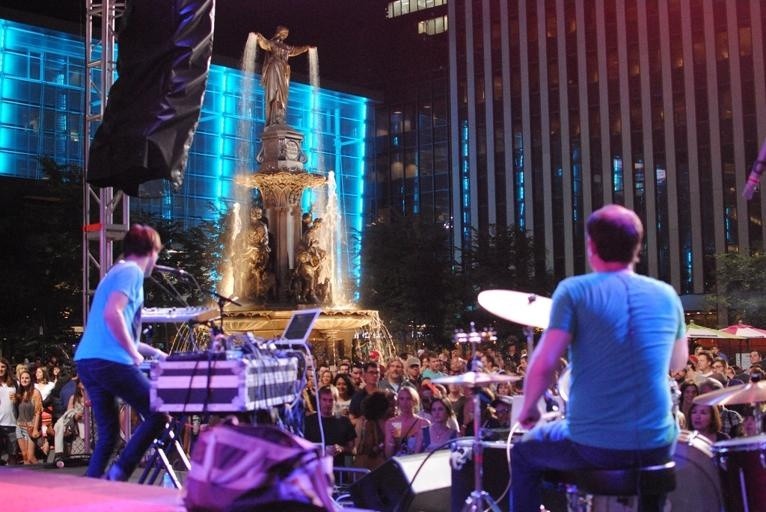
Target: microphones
[155,265,184,276]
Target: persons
[1,358,97,467]
[74,225,171,482]
[296,337,572,490]
[667,342,766,443]
[256,24,311,128]
[113,396,209,478]
[222,207,345,310]
[508,204,689,512]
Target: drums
[711,434,766,511]
[452,440,514,512]
[585,430,726,511]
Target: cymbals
[692,379,766,407]
[432,370,522,384]
[477,288,552,331]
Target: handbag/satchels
[184,416,344,512]
[27,427,42,439]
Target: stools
[548,461,677,512]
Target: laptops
[273,308,321,349]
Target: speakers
[349,449,456,511]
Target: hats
[407,358,420,367]
[491,395,512,407]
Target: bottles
[163,471,175,489]
